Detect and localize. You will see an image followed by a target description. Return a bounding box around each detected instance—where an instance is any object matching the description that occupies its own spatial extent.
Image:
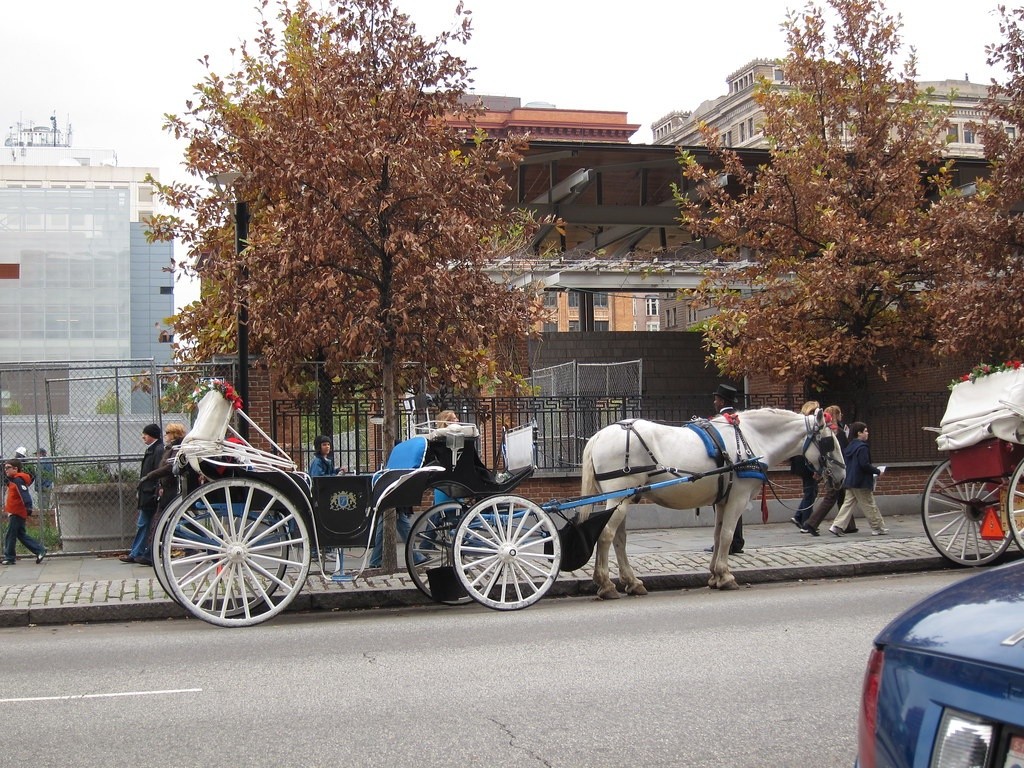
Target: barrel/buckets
[426,543,459,601]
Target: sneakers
[0,560,16,565]
[829,524,846,536]
[36,548,47,564]
[872,527,889,534]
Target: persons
[133,423,201,567]
[829,422,890,537]
[118,423,183,562]
[368,438,432,569]
[802,406,858,537]
[0,447,54,565]
[704,384,745,555]
[790,401,821,533]
[417,410,470,558]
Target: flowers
[179,376,244,410]
[946,360,1020,391]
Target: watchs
[307,435,346,563]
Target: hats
[33,448,47,456]
[712,384,738,403]
[143,424,160,439]
[15,447,27,457]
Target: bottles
[337,469,345,477]
[495,473,505,483]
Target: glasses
[166,431,170,434]
[6,466,13,470]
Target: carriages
[920,358,1024,567]
[149,376,848,628]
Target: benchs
[371,436,428,507]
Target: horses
[579,408,847,601]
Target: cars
[852,556,1024,768]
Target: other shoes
[848,528,859,533]
[791,515,803,528]
[134,556,152,565]
[801,526,806,533]
[704,547,712,551]
[119,555,134,561]
[803,522,820,535]
[312,552,336,562]
